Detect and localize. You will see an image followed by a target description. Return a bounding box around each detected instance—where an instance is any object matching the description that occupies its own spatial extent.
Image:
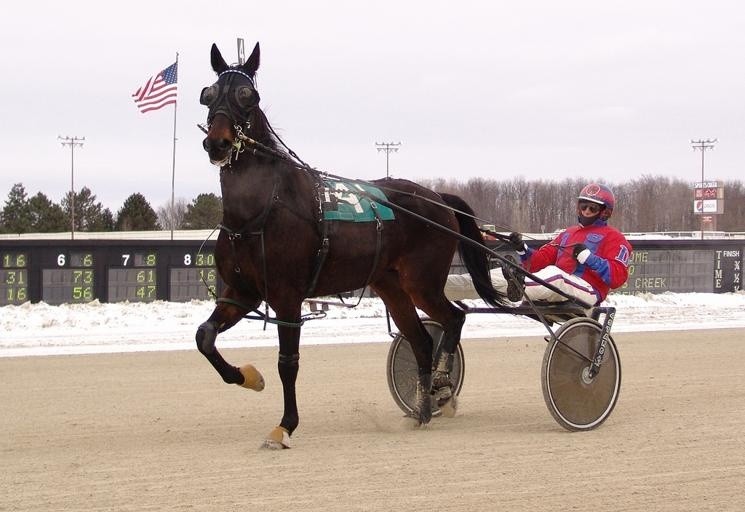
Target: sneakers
[500,254,527,303]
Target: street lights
[373,141,402,180]
[58,135,89,239]
[688,137,721,238]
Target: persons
[446,182,634,325]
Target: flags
[131,61,176,115]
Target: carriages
[187,40,625,453]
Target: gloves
[572,242,592,265]
[508,231,529,255]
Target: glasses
[579,202,602,214]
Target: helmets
[575,182,618,223]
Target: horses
[196,42,515,450]
